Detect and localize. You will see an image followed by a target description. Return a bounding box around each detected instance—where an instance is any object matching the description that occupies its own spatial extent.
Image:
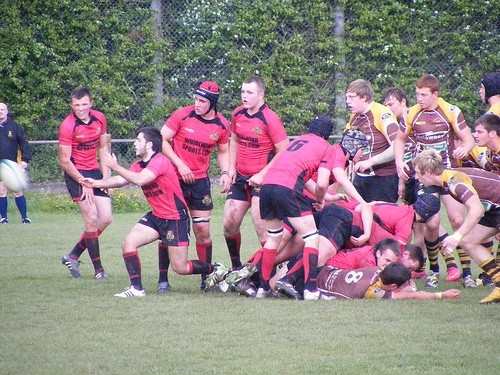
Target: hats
[480,72,500,104]
[413,193,440,222]
[340,129,368,160]
[194,81,220,114]
[308,114,333,140]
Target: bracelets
[452,231,462,242]
[221,171,230,178]
[436,293,442,300]
[317,200,325,204]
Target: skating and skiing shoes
[445,267,461,282]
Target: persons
[474,71,500,285]
[158,80,232,294]
[202,75,475,301]
[79,127,225,299]
[414,150,500,303]
[0,103,32,224]
[58,86,113,279]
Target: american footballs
[0,159,28,193]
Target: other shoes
[0,218,8,223]
[21,218,31,224]
[202,261,305,300]
[156,281,171,294]
[287,254,303,270]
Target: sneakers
[474,272,493,287]
[411,269,427,280]
[425,270,440,288]
[62,253,85,279]
[114,285,145,297]
[479,287,500,304]
[303,288,337,302]
[463,272,477,288]
[94,271,107,279]
